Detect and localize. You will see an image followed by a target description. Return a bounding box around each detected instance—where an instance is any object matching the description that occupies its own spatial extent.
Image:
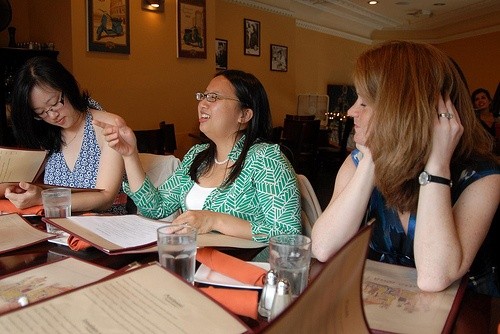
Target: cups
[270,235,313,297]
[42,188,73,238]
[156,224,197,288]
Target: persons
[0,56,126,212]
[471,88,500,143]
[311,40,500,292]
[92,71,302,262]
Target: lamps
[142,0,164,12]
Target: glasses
[34,90,65,121]
[195,92,242,102]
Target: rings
[440,113,452,119]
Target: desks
[0,211,499,334]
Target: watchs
[419,171,451,187]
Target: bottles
[257,268,293,320]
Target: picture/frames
[244,18,260,56]
[216,38,228,70]
[176,0,207,59]
[86,0,130,54]
[270,44,288,72]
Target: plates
[47,236,68,246]
[194,262,271,289]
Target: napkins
[196,247,268,287]
[199,288,258,320]
[0,200,44,216]
[68,234,91,251]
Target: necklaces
[215,159,228,164]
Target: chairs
[273,115,353,236]
[127,122,182,222]
